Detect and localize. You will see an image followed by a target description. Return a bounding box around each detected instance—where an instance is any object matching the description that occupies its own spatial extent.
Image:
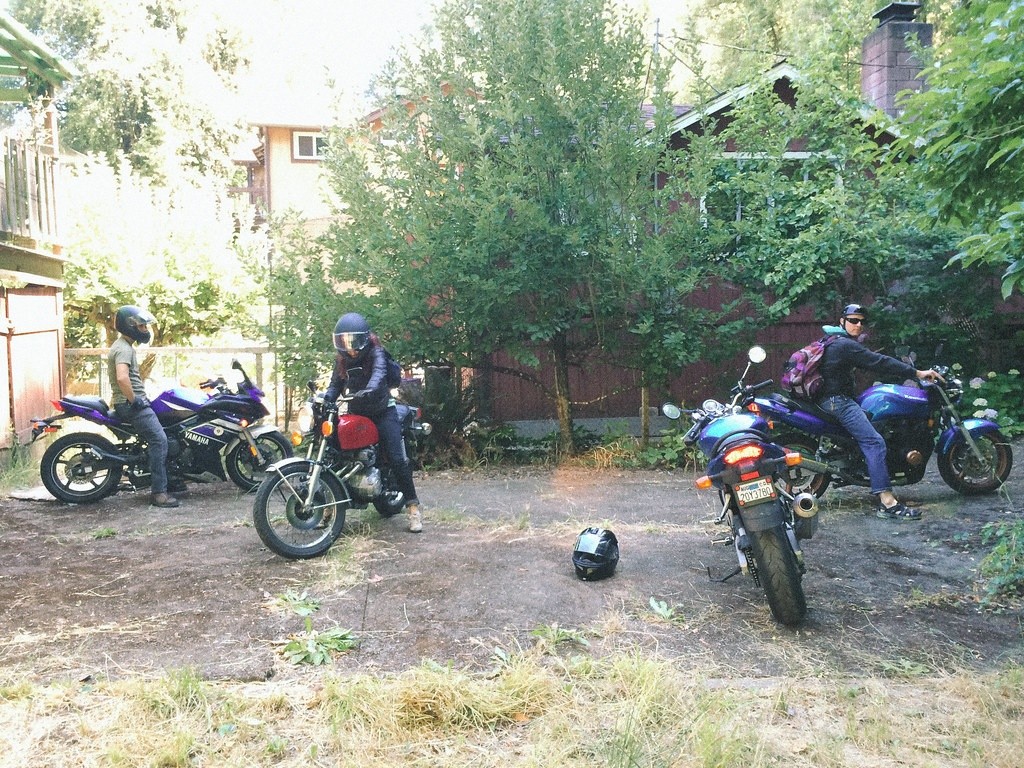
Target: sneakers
[409,514,422,532]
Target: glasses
[844,317,866,325]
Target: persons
[324,313,423,532]
[109,306,180,507]
[814,304,948,520]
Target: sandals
[877,501,921,520]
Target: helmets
[333,312,371,365]
[115,305,158,347]
[843,303,868,317]
[572,527,619,580]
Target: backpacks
[782,334,847,402]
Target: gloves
[126,397,151,411]
[355,388,374,405]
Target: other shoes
[168,480,189,491]
[152,493,179,508]
[314,510,332,529]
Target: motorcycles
[746,345,1013,499]
[252,367,432,559]
[662,345,818,626]
[30,358,293,503]
